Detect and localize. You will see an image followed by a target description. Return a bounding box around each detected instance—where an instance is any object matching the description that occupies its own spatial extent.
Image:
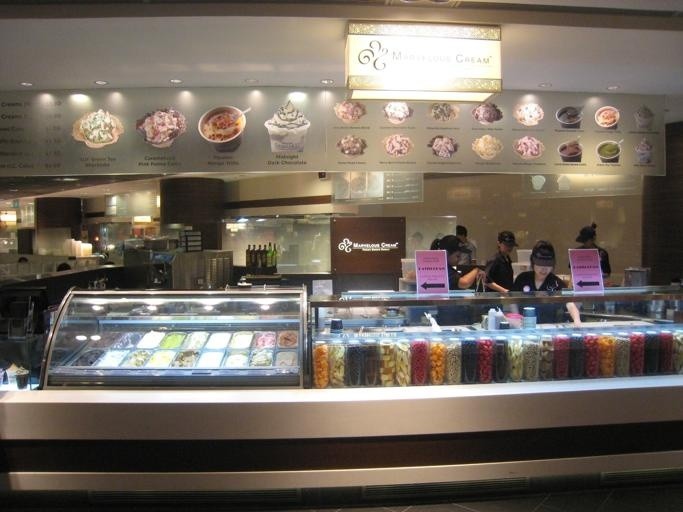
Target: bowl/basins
[198,107,247,153]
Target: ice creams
[335,102,654,162]
[79,105,244,143]
[269,100,308,153]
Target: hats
[532,252,555,266]
[576,233,594,242]
[498,236,518,245]
[440,238,472,254]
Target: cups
[500,322,510,331]
[556,104,582,130]
[0,367,29,392]
[596,140,622,164]
[331,320,344,336]
[264,118,311,155]
[635,143,655,163]
[72,240,93,256]
[595,105,620,131]
[558,140,583,162]
[635,108,656,129]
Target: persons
[429,221,613,331]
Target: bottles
[312,331,683,388]
[245,242,278,275]
[488,309,504,331]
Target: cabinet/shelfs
[38,284,310,391]
[309,284,683,391]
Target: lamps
[343,20,503,103]
